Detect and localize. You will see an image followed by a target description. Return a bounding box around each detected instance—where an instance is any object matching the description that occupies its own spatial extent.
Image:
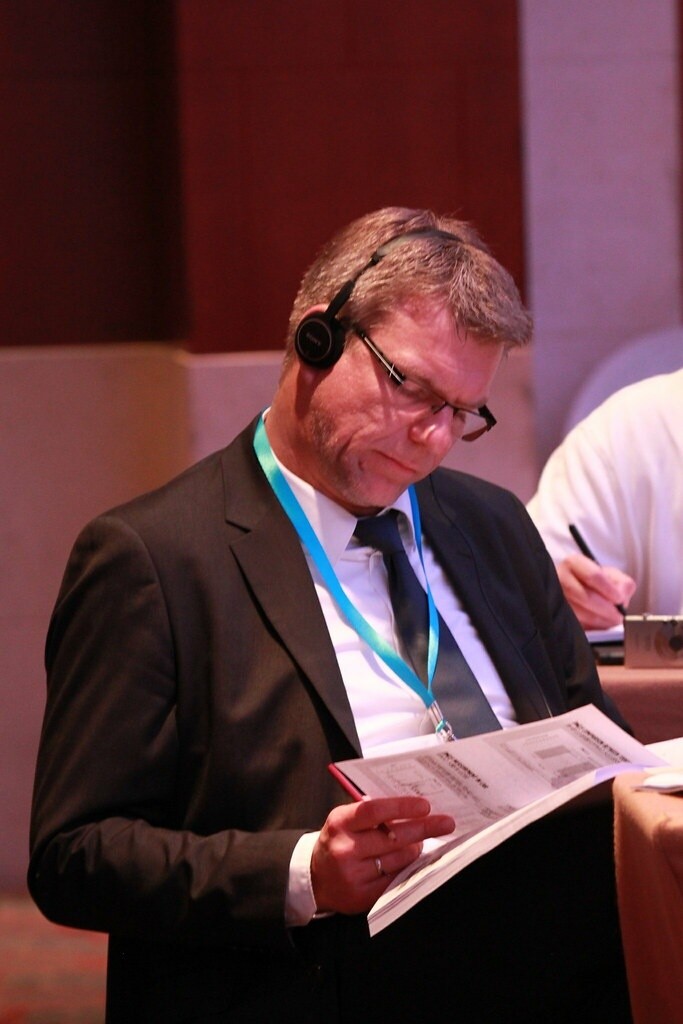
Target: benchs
[0,350,542,1024]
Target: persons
[27,207,683,1024]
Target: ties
[353,508,504,739]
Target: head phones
[297,230,462,366]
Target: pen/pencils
[569,521,627,615]
[326,759,396,844]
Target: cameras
[625,614,683,670]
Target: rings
[374,857,385,877]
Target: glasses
[350,321,497,442]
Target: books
[326,699,676,942]
